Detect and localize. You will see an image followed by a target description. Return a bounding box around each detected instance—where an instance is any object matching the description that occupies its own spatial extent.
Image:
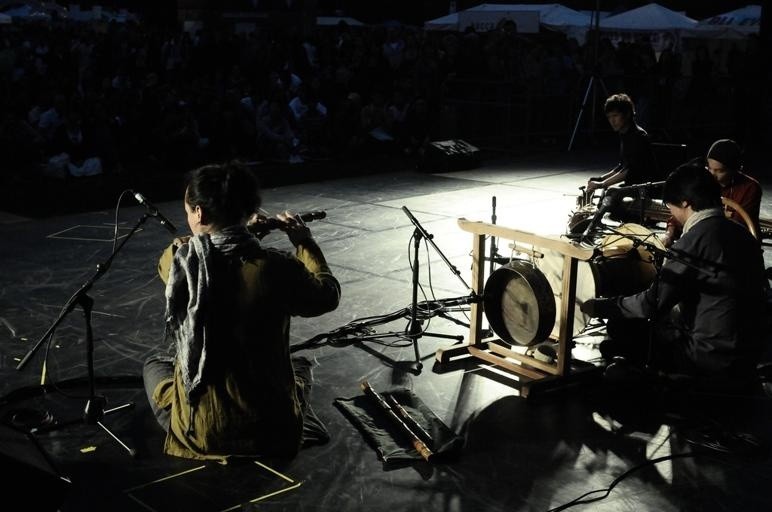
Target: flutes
[248,208,326,234]
[360,379,433,463]
[388,392,433,444]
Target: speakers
[424,139,480,173]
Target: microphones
[135,194,177,234]
[583,196,614,236]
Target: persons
[599,93,652,191]
[3,2,491,183]
[495,18,537,145]
[578,165,772,391]
[143,163,341,464]
[537,31,756,145]
[705,138,763,245]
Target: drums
[536,224,666,338]
[483,259,556,346]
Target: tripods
[355,258,462,371]
[26,319,139,458]
